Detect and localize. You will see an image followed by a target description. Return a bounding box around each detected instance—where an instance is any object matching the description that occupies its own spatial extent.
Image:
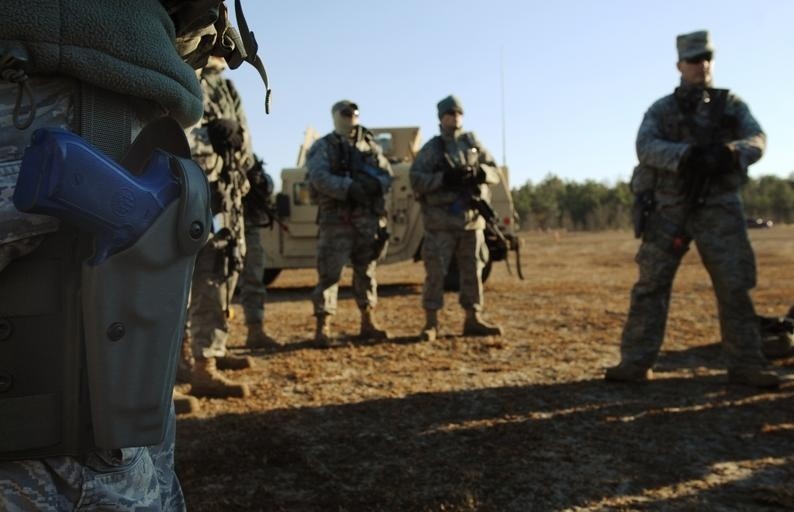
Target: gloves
[350,173,379,206]
[679,144,734,203]
[442,165,486,187]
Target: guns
[433,151,510,250]
[13,123,180,267]
[245,186,289,233]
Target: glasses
[443,111,463,116]
[341,107,360,117]
[686,51,712,64]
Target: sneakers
[727,368,779,387]
[605,358,653,381]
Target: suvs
[255,126,529,290]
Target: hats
[676,29,713,62]
[437,95,464,121]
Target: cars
[743,214,774,230]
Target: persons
[604,31,780,387]
[176,288,250,383]
[409,95,502,341]
[304,101,394,348]
[172,55,254,414]
[0,0,220,512]
[237,154,281,347]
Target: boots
[173,337,254,414]
[246,322,283,353]
[359,307,391,338]
[464,307,501,336]
[421,309,443,342]
[315,314,333,340]
